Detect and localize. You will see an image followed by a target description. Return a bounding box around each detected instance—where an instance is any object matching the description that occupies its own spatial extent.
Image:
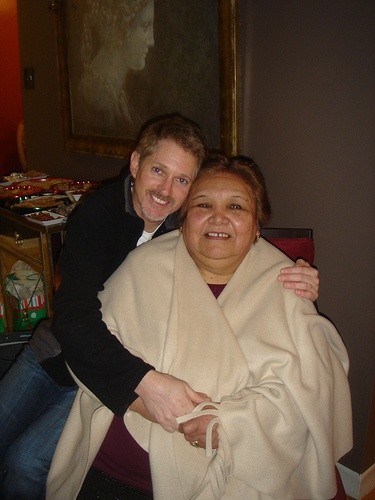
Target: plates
[65,190,81,201]
[10,199,63,209]
[25,211,64,226]
[4,172,48,182]
[34,194,68,199]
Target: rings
[192,441,199,447]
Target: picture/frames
[53,0,241,162]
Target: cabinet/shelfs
[0,170,67,334]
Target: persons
[46,158,354,500]
[1,117,319,500]
